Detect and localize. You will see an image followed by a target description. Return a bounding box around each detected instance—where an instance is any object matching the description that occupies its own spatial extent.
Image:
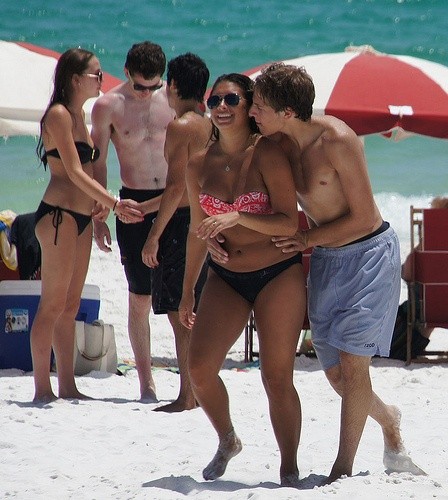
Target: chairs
[406,205,448,363]
[245,209,317,363]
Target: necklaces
[226,165,230,172]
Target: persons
[205,63,402,487]
[403,196,448,338]
[90,42,176,402]
[179,73,307,488]
[30,47,144,405]
[142,52,216,414]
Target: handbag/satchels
[73,318,118,374]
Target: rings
[213,222,216,224]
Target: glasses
[207,93,246,109]
[127,69,164,91]
[83,71,104,84]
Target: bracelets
[113,201,118,211]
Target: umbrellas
[202,45,448,143]
[0,40,124,140]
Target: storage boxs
[0,280,100,373]
